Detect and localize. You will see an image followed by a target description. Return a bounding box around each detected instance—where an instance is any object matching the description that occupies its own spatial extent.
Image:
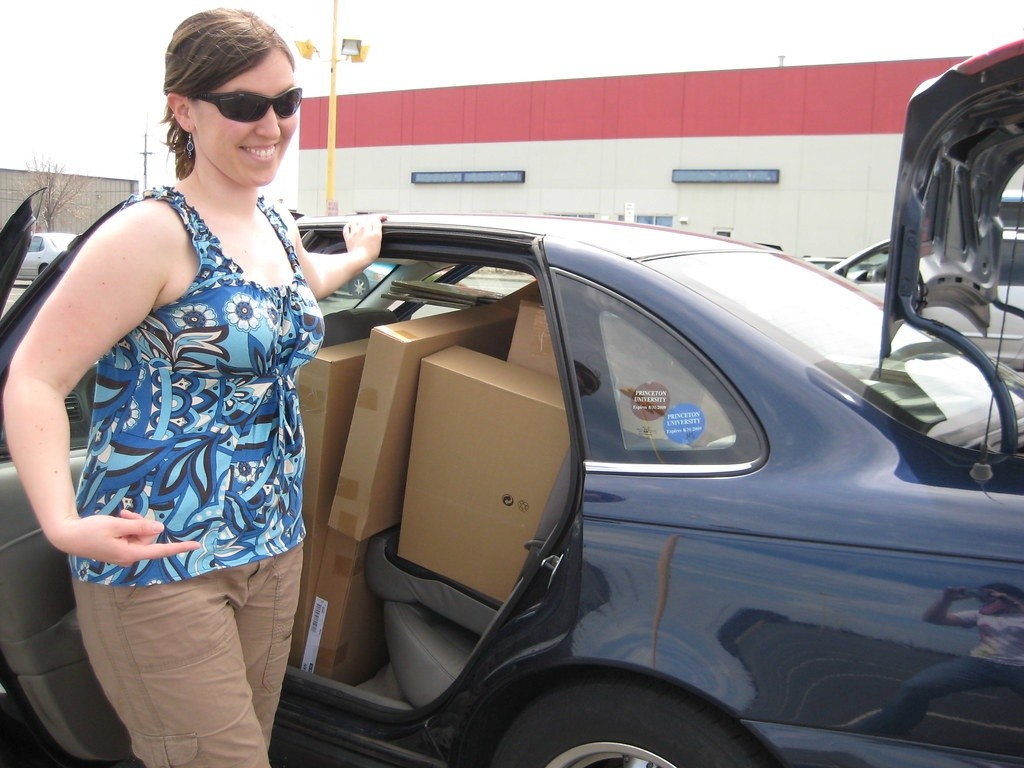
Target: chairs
[365,531,505,707]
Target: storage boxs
[287,275,730,688]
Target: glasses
[187,86,303,122]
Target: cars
[13,231,79,279]
[331,262,396,301]
[801,191,1023,371]
[2,28,1024,768]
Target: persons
[2,4,388,768]
